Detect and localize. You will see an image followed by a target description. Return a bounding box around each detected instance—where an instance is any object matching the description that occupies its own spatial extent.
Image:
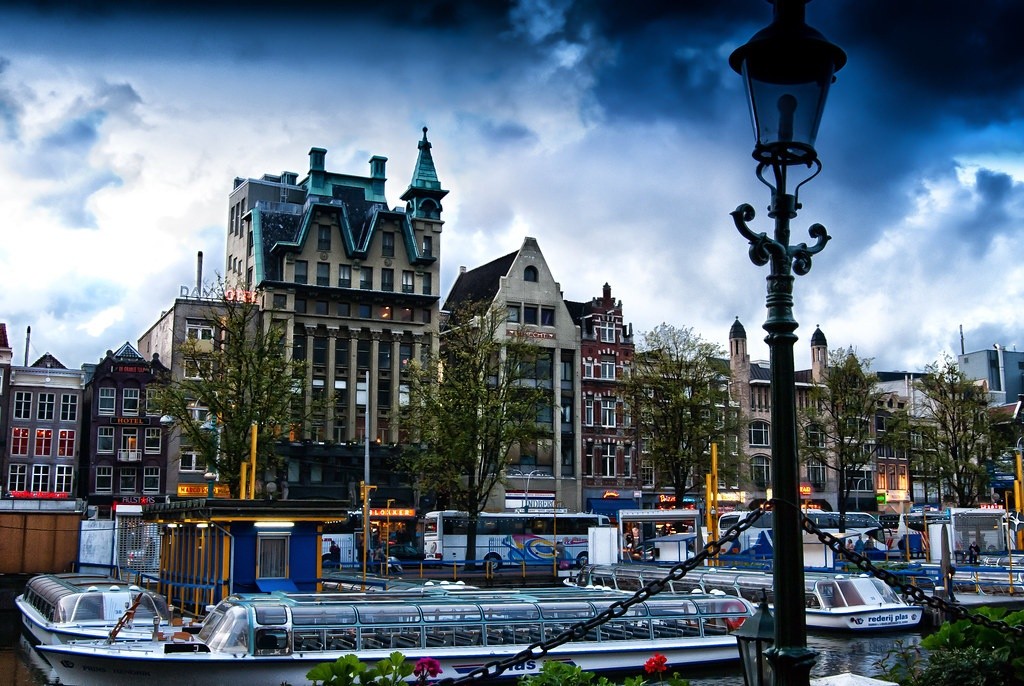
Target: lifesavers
[720,599,747,631]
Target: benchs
[292,619,730,651]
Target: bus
[718,509,893,561]
[424,510,611,571]
[878,513,945,557]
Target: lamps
[196,523,209,528]
[167,522,177,528]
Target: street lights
[844,477,872,511]
[506,469,548,514]
[730,0,849,686]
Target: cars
[384,545,436,568]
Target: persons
[847,535,874,551]
[358,540,388,571]
[626,531,632,552]
[969,541,980,563]
[940,550,960,603]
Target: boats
[33,585,758,686]
[322,569,480,592]
[907,556,1024,594]
[562,563,924,637]
[15,573,183,653]
